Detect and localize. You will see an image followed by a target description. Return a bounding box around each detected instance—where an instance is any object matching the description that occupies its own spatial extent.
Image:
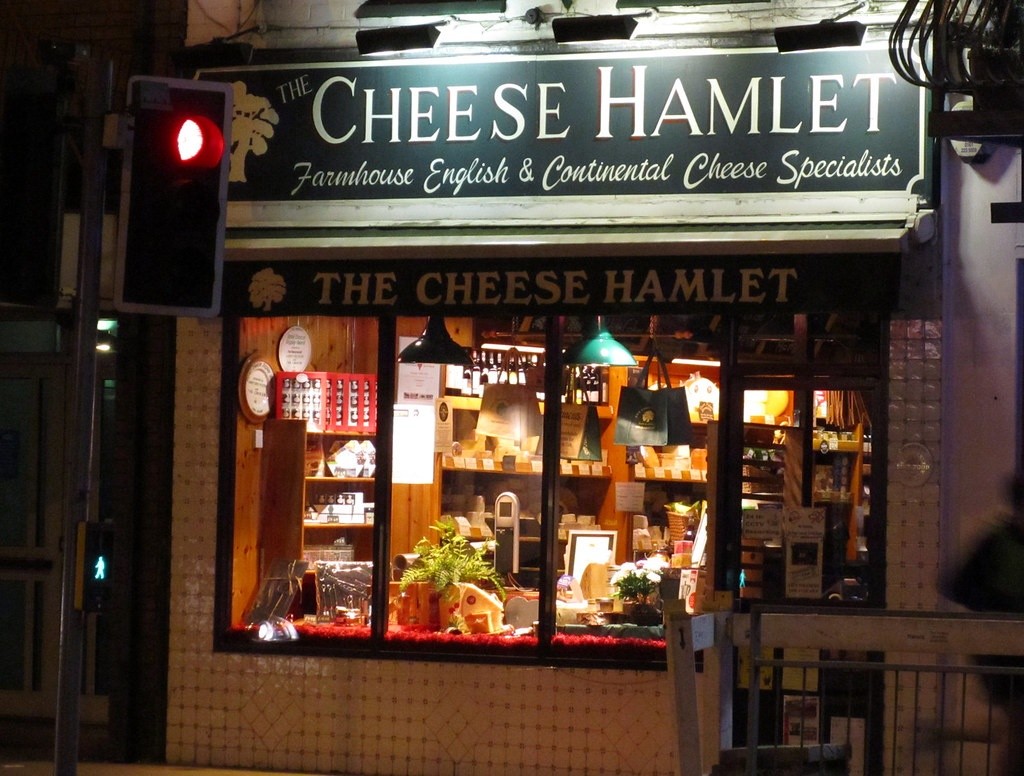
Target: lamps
[355,19,452,58]
[396,315,475,367]
[562,317,640,369]
[775,3,871,55]
[551,11,656,44]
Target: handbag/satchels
[614,348,693,446]
[535,365,602,461]
[476,348,542,441]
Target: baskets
[667,512,689,539]
[742,465,784,494]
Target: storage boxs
[674,540,693,554]
[677,569,706,616]
[671,553,692,569]
[656,452,692,470]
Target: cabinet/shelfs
[256,347,871,621]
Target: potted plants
[399,512,507,631]
[602,568,664,627]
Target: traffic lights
[115,74,232,318]
[76,522,117,613]
